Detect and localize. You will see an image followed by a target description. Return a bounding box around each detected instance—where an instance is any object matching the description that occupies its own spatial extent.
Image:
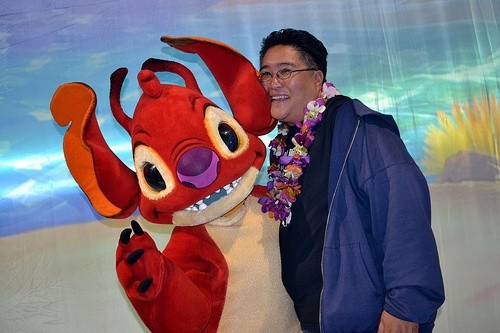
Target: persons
[259,27,446,333]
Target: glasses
[261,68,317,81]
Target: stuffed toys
[49,36,305,333]
